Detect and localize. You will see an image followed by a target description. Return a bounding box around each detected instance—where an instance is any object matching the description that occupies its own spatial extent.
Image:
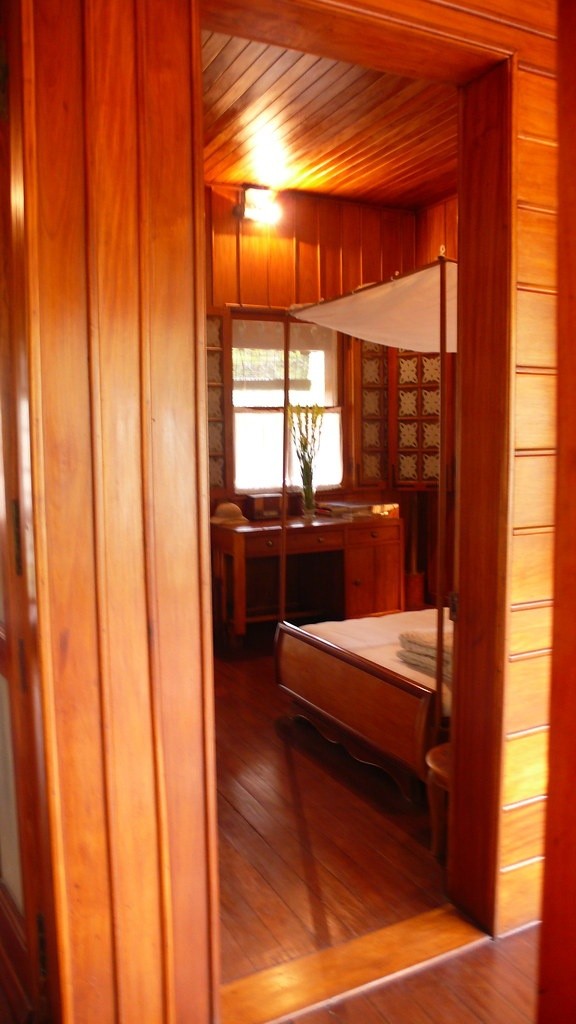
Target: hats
[211,502,251,526]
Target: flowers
[287,402,325,509]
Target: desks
[216,510,404,635]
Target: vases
[301,485,317,518]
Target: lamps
[230,187,277,223]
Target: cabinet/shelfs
[344,525,404,621]
[209,185,417,309]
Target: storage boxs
[245,493,283,519]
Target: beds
[272,606,455,802]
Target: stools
[425,743,450,861]
